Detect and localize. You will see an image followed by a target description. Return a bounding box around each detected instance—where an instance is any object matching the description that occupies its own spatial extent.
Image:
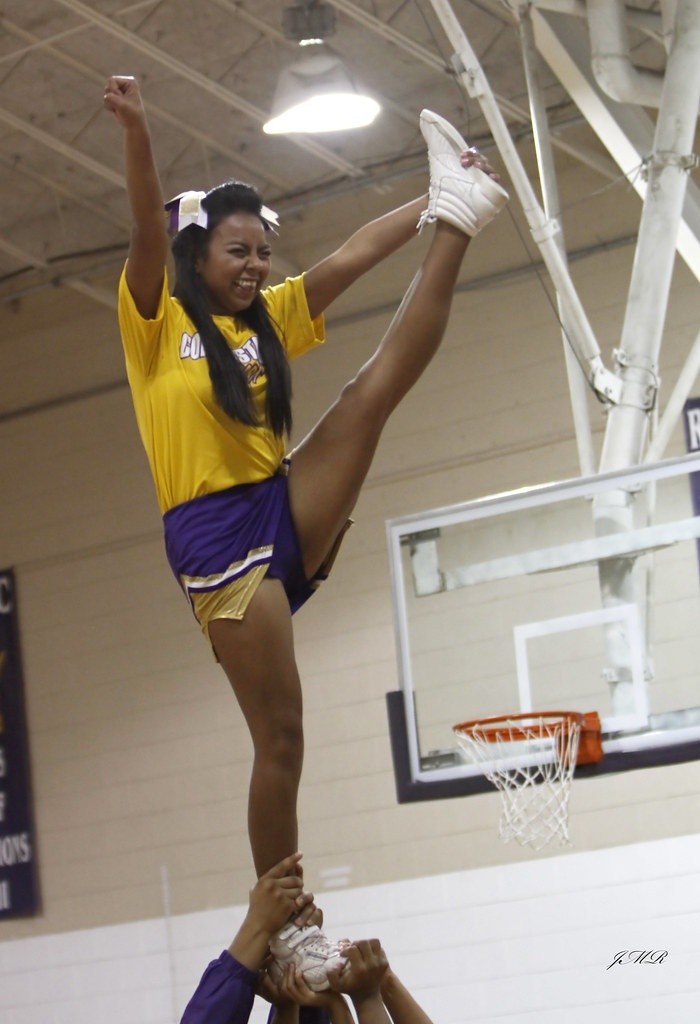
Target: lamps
[263,1,383,137]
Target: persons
[103,76,511,992]
[326,938,433,1024]
[257,953,354,1024]
[180,851,323,1024]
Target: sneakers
[264,922,359,991]
[419,109,511,237]
